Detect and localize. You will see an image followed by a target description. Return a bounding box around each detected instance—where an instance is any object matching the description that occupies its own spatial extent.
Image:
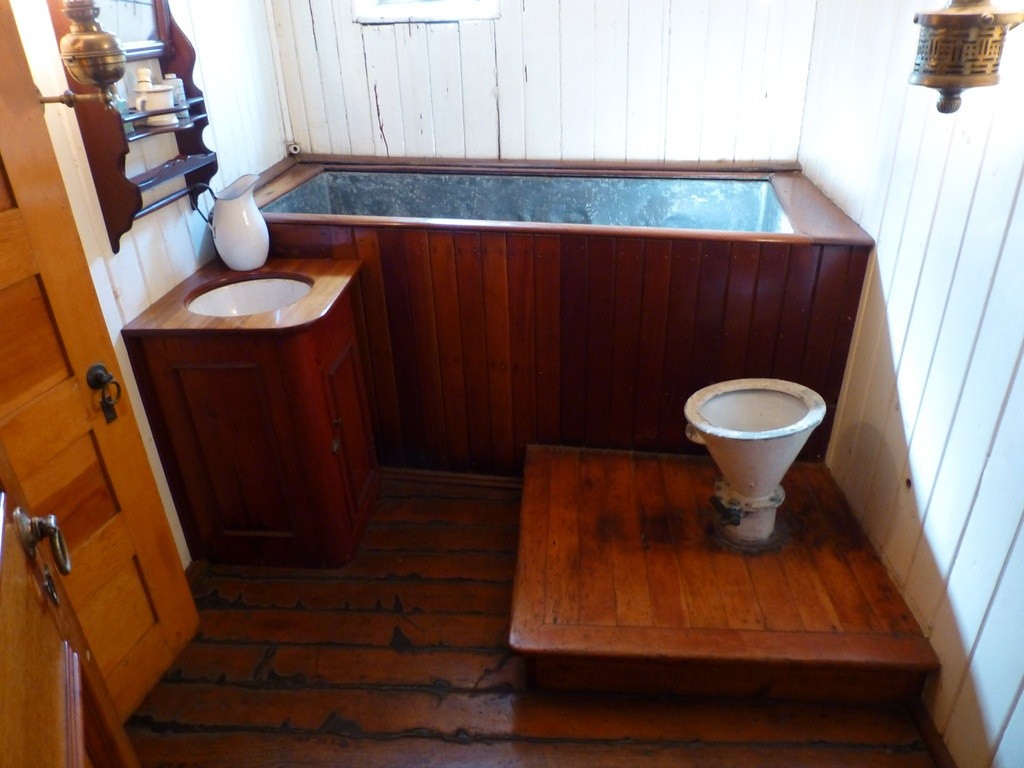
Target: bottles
[162,73,190,117]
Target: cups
[133,84,179,126]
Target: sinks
[183,271,316,318]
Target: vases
[191,170,270,271]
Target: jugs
[187,173,270,272]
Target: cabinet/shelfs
[142,223,873,568]
[50,0,218,254]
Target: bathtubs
[206,152,878,482]
